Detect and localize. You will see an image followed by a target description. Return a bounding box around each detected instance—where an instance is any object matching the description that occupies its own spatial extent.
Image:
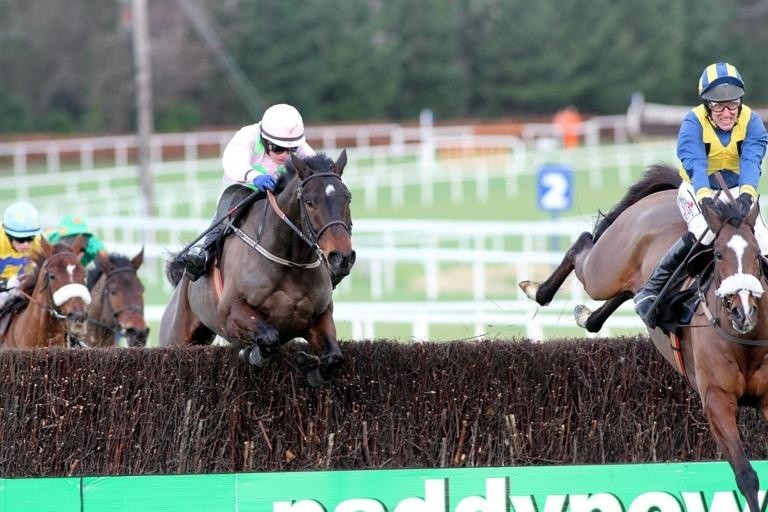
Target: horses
[517,165,768,512]
[77,244,151,350]
[0,232,91,351]
[157,146,357,390]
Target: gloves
[698,193,753,228]
[253,174,277,193]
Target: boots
[633,232,701,328]
[184,220,228,281]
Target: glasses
[707,100,742,111]
[268,144,298,153]
[14,237,34,243]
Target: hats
[58,214,94,239]
[698,61,745,94]
[1,201,42,237]
[260,103,305,147]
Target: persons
[633,62,768,330]
[0,201,45,292]
[184,102,318,282]
[552,103,582,148]
[45,214,109,272]
